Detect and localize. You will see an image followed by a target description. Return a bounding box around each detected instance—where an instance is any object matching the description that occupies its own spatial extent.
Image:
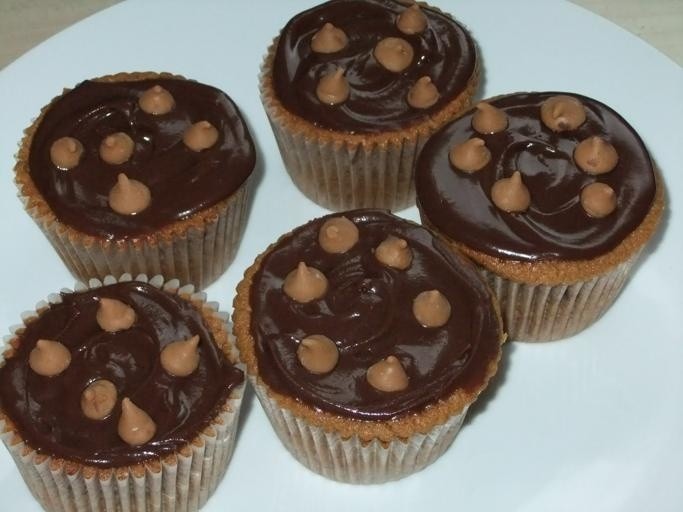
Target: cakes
[231,207,508,486]
[259,0,483,214]
[415,90,666,344]
[0,272,247,512]
[11,71,264,294]
